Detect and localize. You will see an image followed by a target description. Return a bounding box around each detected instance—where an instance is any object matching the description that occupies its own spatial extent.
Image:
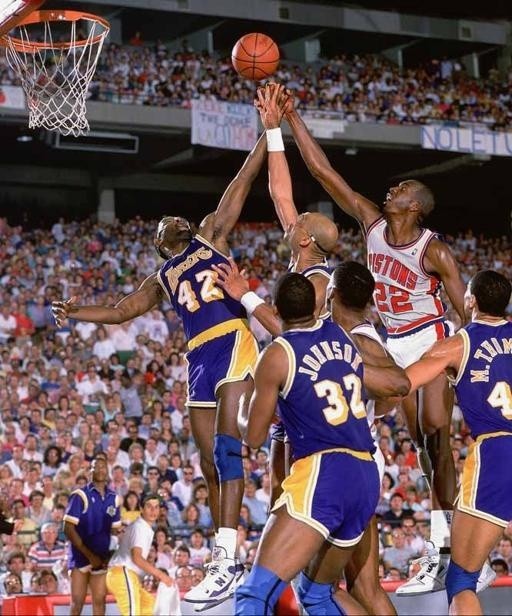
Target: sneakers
[394,539,452,597]
[183,545,250,612]
[475,561,497,594]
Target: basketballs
[231,32,280,79]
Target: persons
[0,27,511,616]
